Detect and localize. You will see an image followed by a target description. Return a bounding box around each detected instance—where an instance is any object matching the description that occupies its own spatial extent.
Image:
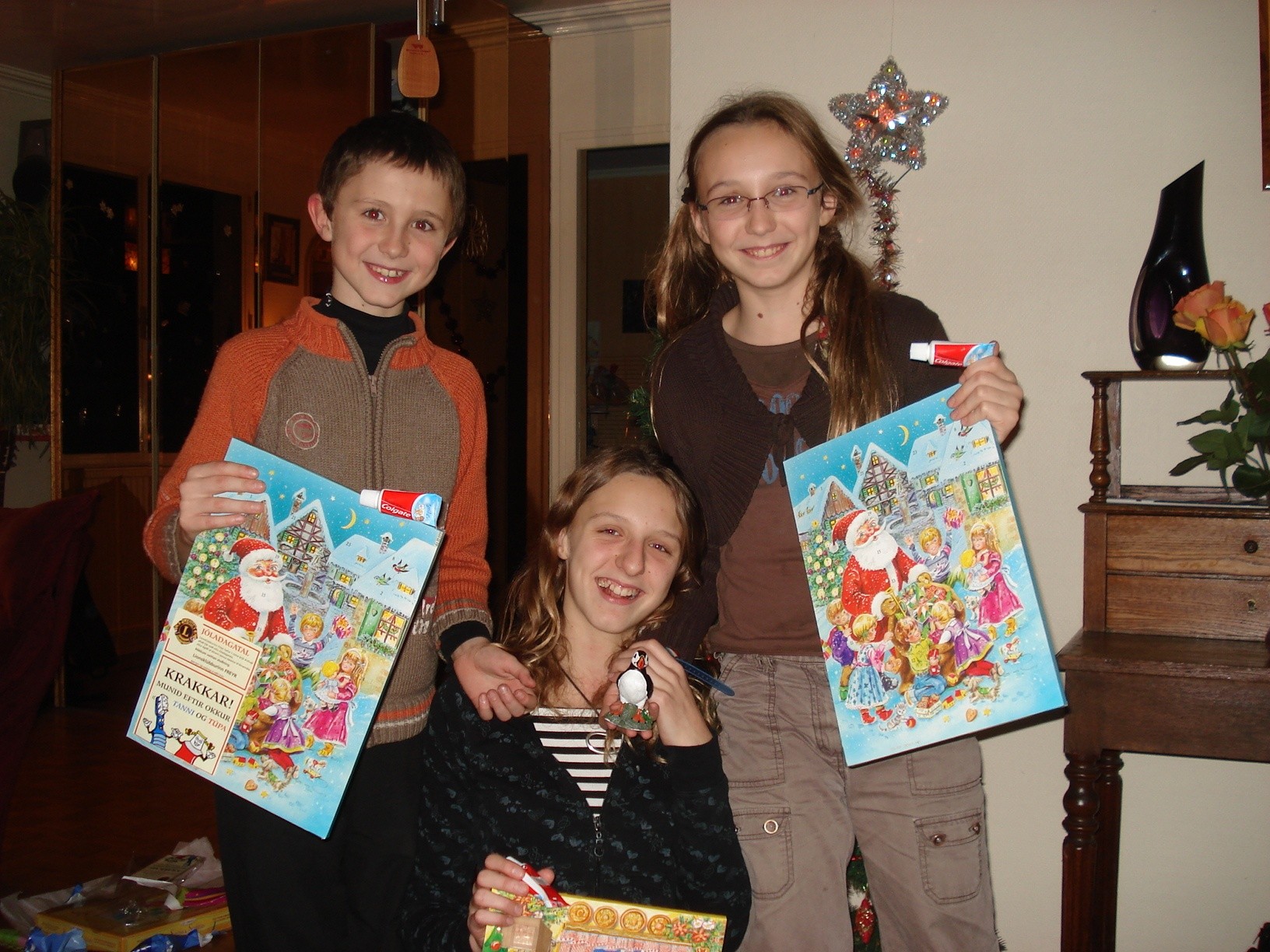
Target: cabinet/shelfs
[1080,370,1270,646]
[47,24,375,710]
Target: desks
[1056,627,1270,952]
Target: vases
[1127,159,1213,371]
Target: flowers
[1177,279,1270,501]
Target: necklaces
[543,635,625,739]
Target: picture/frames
[259,213,301,285]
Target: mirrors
[156,179,244,452]
[59,162,143,457]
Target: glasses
[698,181,826,220]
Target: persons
[141,107,538,951]
[380,441,753,952]
[635,89,1026,951]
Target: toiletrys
[910,341,996,368]
[357,488,443,529]
